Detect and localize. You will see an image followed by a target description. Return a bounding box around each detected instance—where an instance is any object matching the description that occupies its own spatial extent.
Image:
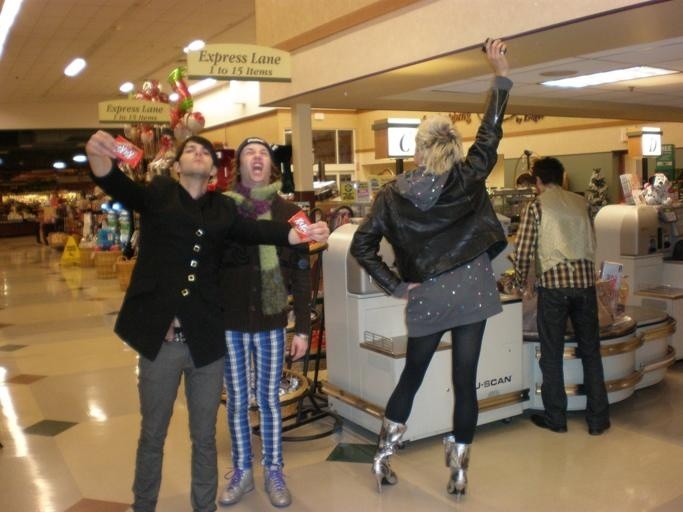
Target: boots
[371,416,408,492]
[444,434,473,503]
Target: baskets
[286,305,320,350]
[221,368,309,429]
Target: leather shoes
[531,413,566,432]
[588,420,610,434]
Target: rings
[500,50,505,54]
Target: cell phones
[482,38,507,55]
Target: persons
[588,167,608,206]
[219,137,312,509]
[50,191,88,235]
[510,156,610,436]
[517,172,537,188]
[85,130,331,512]
[349,37,514,501]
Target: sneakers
[218,467,254,505]
[264,465,291,507]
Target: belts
[165,332,187,343]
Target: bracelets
[297,333,311,340]
